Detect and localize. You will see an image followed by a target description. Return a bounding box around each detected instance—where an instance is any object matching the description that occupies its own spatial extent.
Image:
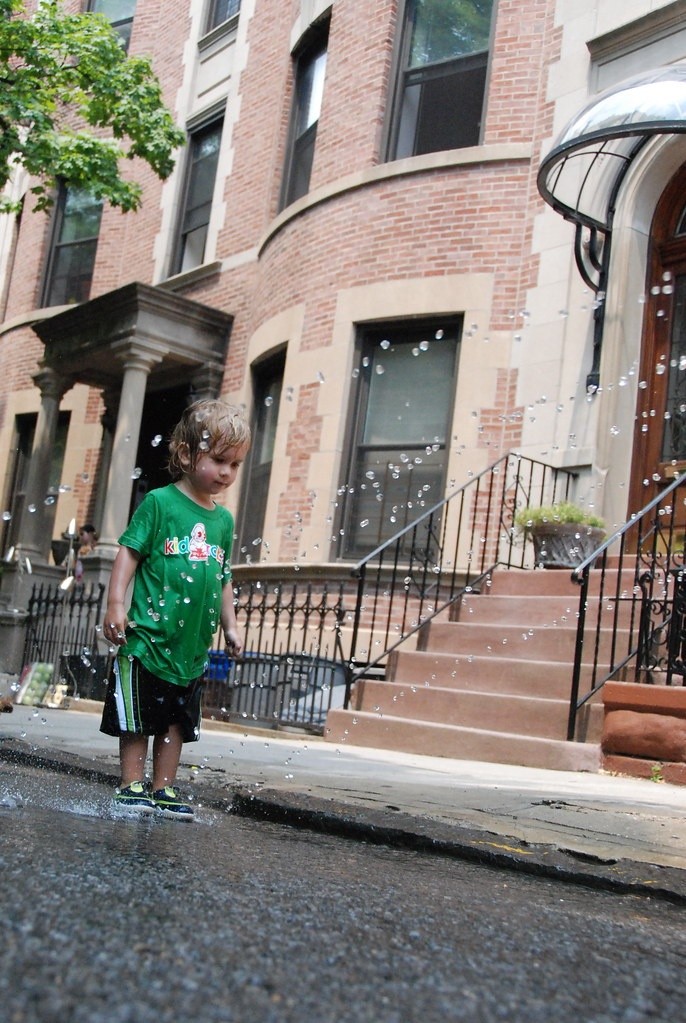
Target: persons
[99,400,255,822]
[76,523,98,560]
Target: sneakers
[144,785,195,822]
[112,779,157,816]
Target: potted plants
[514,505,605,567]
